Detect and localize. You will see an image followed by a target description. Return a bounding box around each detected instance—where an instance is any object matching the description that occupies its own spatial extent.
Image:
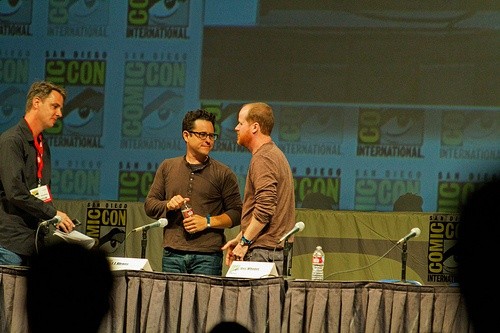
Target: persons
[144,108,243,275]
[220,102,295,275]
[24,241,116,333]
[0,81,76,254]
[454,173,500,333]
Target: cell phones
[59,219,82,234]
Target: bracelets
[242,236,250,244]
[206,215,211,228]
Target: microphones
[277,222,305,244]
[131,218,168,232]
[40,216,61,227]
[396,228,421,245]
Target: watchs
[240,236,252,247]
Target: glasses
[188,131,218,140]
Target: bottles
[312,246,324,280]
[181,200,198,234]
